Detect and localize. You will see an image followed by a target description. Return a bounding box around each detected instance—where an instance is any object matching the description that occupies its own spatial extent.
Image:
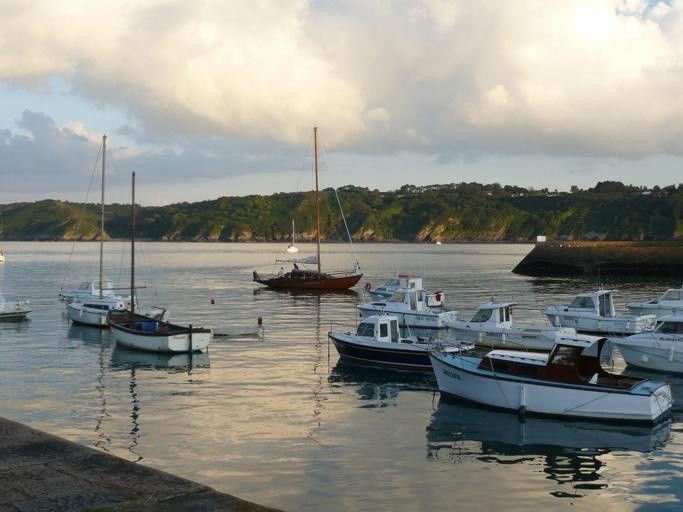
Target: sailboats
[251,126,364,292]
[56,132,215,356]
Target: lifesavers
[366,283,371,291]
